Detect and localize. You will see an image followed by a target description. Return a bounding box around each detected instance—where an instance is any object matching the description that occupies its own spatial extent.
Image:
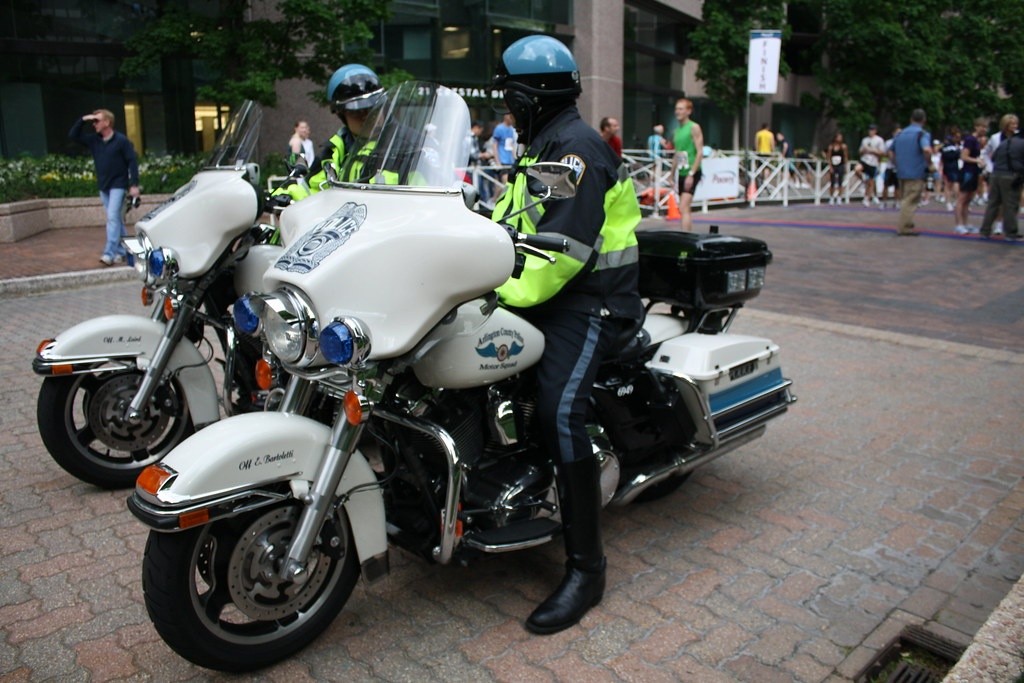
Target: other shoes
[898,228,920,236]
[114,254,126,263]
[893,203,899,211]
[982,233,987,239]
[99,255,114,266]
[916,193,991,213]
[862,197,870,207]
[954,224,968,235]
[872,196,879,205]
[910,224,915,228]
[837,196,841,205]
[994,221,1002,234]
[884,203,887,210]
[966,223,979,233]
[829,197,834,206]
[1005,234,1024,242]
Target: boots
[526,454,607,635]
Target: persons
[777,133,792,185]
[980,131,1024,241]
[756,124,776,187]
[268,62,456,248]
[647,125,669,185]
[792,148,819,189]
[922,114,1019,235]
[288,120,317,171]
[82,109,140,266]
[887,109,931,234]
[826,132,850,204]
[464,119,490,198]
[491,112,522,206]
[596,116,623,162]
[486,36,645,638]
[854,125,902,209]
[669,98,703,231]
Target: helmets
[486,35,582,96]
[327,63,388,113]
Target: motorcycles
[27,98,292,491]
[121,84,800,677]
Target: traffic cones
[664,191,682,221]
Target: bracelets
[688,171,694,177]
